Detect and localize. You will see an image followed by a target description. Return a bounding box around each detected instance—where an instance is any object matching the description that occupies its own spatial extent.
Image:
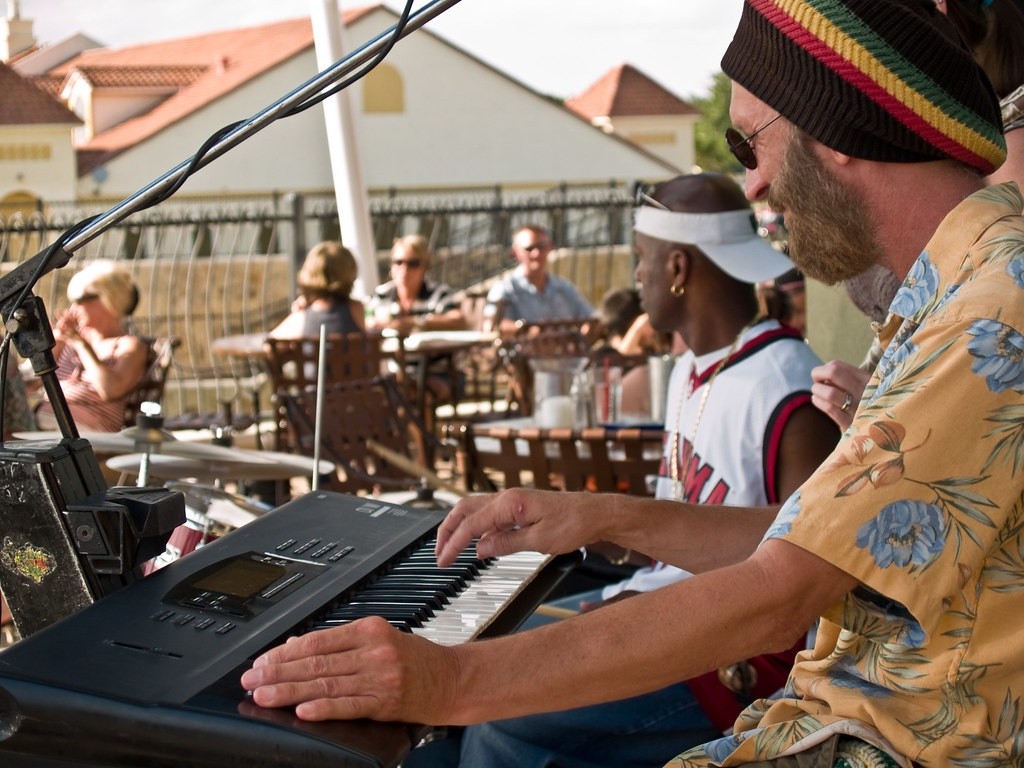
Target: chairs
[123,335,186,433]
[266,288,667,497]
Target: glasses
[525,242,545,252]
[397,259,420,267]
[726,116,783,170]
[76,294,98,303]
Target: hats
[720,1,1006,174]
[297,242,358,292]
[633,204,796,282]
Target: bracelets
[608,548,631,563]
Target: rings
[842,394,851,411]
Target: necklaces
[672,318,758,498]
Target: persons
[458,170,841,768]
[265,241,365,392]
[482,225,597,397]
[242,1,1023,767]
[18,260,150,436]
[774,269,807,331]
[595,288,669,357]
[366,235,467,402]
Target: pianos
[0,486,594,768]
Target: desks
[457,417,659,478]
[210,331,496,491]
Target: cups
[648,354,674,423]
[589,367,623,424]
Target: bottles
[569,373,593,430]
